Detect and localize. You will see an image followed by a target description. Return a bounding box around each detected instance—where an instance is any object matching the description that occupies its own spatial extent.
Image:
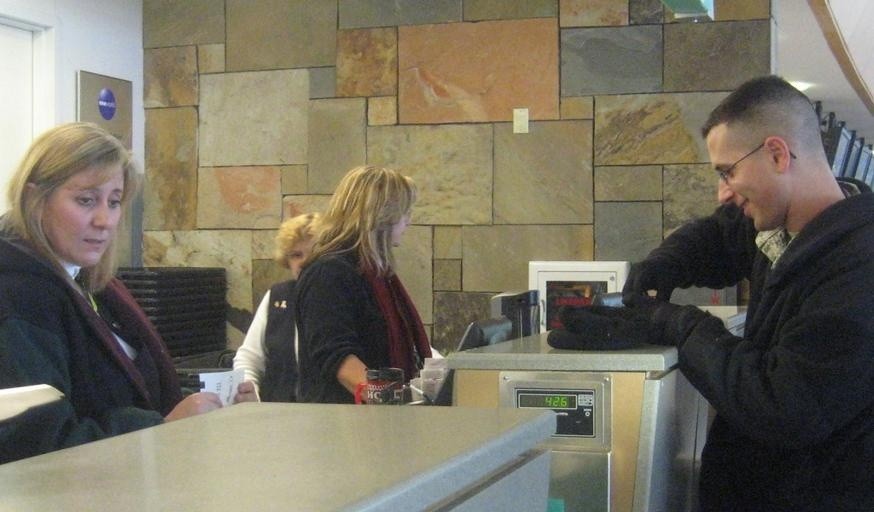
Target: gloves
[620,256,682,303]
[556,293,680,345]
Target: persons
[233,212,323,400]
[0,120,258,466]
[548,77,873,512]
[300,162,433,404]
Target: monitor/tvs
[430,317,513,407]
[526,258,632,334]
[490,288,540,343]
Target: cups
[363,366,406,404]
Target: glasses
[719,143,796,185]
